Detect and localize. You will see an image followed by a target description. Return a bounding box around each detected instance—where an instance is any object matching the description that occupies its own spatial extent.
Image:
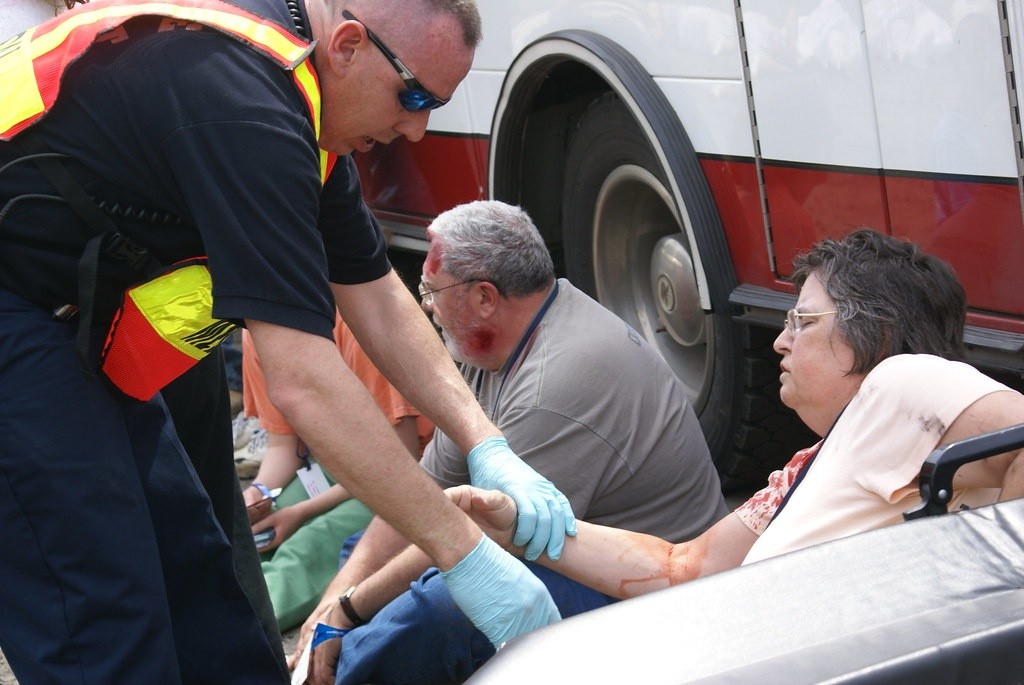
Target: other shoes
[233,428,269,480]
[230,408,262,449]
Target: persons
[1,0,579,685]
[222,202,731,685]
[446,227,1024,599]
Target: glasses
[418,278,507,305]
[783,309,838,337]
[342,5,453,112]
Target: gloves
[469,438,576,564]
[441,529,563,653]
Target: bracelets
[250,483,268,496]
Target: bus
[310,0,1024,496]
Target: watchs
[338,586,364,628]
